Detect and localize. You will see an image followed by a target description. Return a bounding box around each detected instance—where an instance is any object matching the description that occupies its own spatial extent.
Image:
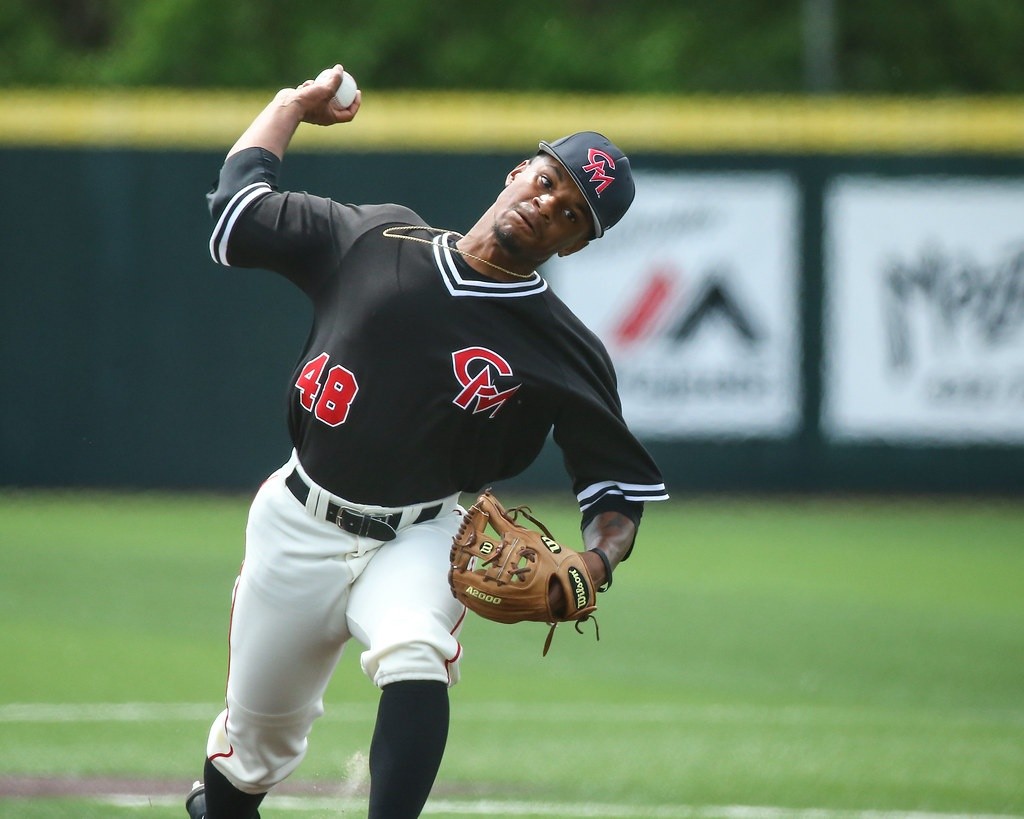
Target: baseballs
[314,69,358,111]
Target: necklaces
[383,225,536,277]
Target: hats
[539,130,635,240]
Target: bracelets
[589,548,612,592]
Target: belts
[285,467,444,541]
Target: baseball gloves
[446,490,599,626]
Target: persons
[185,62,671,819]
